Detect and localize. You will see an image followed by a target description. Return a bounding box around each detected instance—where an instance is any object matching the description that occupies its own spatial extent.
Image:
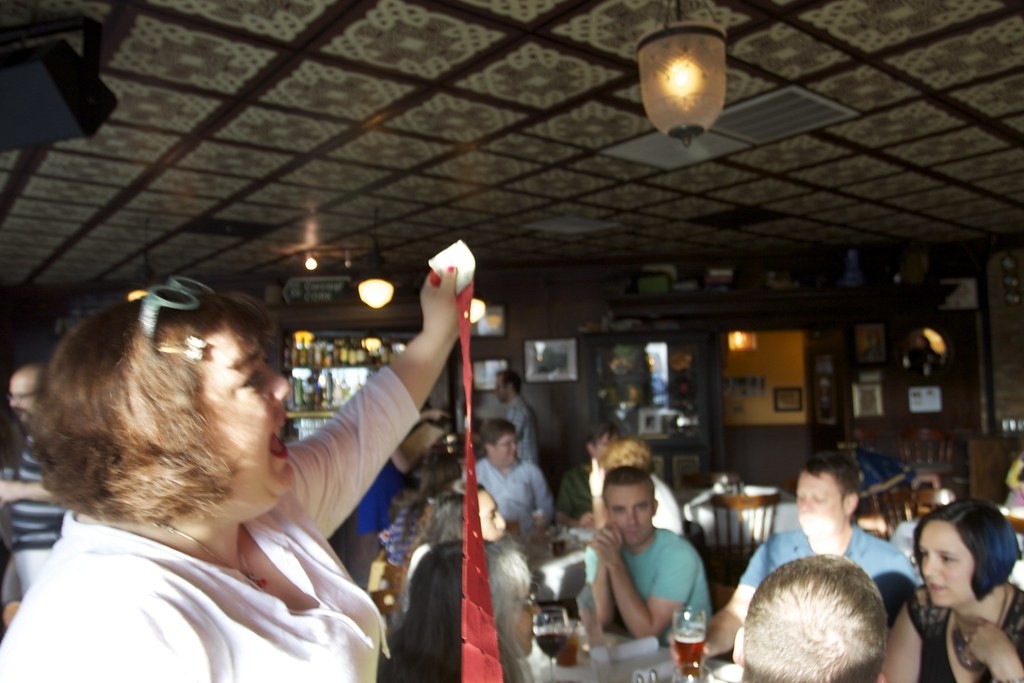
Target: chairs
[852,427,1024,541]
[679,471,779,613]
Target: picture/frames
[469,303,508,339]
[852,383,883,416]
[522,337,579,382]
[471,357,511,393]
[775,388,802,411]
[849,319,891,368]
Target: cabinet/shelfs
[967,433,1024,503]
[581,328,711,489]
[282,329,450,419]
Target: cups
[671,608,706,678]
[550,525,566,555]
[532,511,547,536]
[505,514,520,538]
[556,621,581,666]
[709,663,743,682]
[673,665,714,683]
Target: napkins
[631,658,675,683]
[612,636,659,661]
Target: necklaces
[949,580,1011,678]
[143,523,269,589]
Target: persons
[0,262,474,683]
[359,366,1024,683]
[0,361,74,628]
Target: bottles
[283,335,395,411]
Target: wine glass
[533,607,570,683]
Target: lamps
[0,15,118,151]
[634,0,728,147]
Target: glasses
[138,275,216,342]
[7,390,36,402]
[513,592,536,614]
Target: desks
[528,623,731,683]
[530,525,592,599]
